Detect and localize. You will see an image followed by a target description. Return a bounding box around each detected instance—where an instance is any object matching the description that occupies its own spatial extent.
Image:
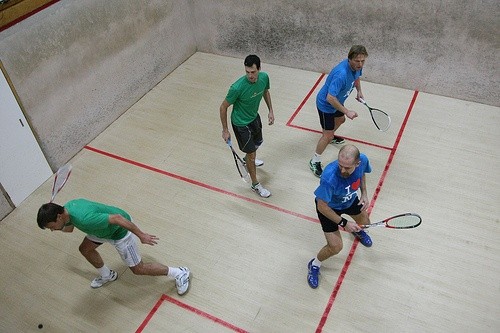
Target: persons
[220,55,274,198]
[310,45,368,177]
[307,144,373,289]
[37,199,190,295]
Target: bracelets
[338,217,347,227]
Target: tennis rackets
[357,213,421,229]
[227,138,250,184]
[355,96,391,133]
[48,163,73,202]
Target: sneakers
[307,258,320,288]
[329,136,345,143]
[309,158,323,177]
[250,182,271,198]
[90,269,117,288]
[243,157,263,167]
[174,267,190,295]
[352,229,372,247]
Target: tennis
[38,323,44,330]
